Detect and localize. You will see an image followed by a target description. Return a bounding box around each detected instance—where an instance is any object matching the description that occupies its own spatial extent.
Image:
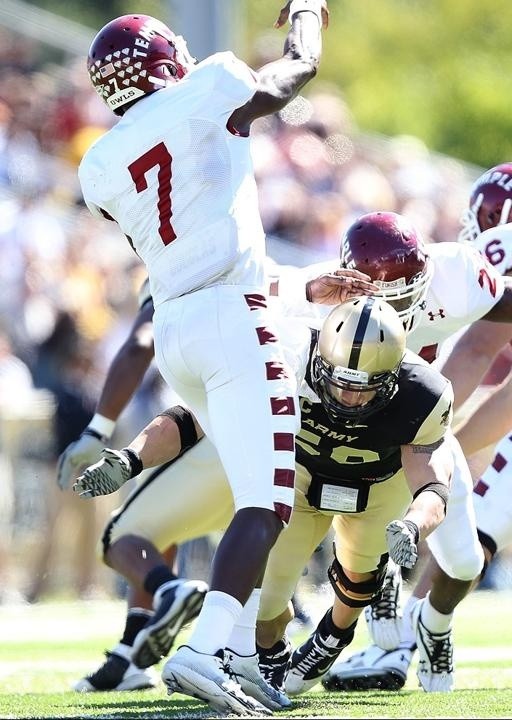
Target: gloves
[386,518,419,570]
[72,448,133,501]
[56,428,109,490]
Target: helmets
[308,297,407,430]
[342,211,430,334]
[459,163,512,287]
[86,15,197,115]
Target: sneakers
[411,598,455,691]
[161,636,296,715]
[286,608,354,692]
[322,644,417,690]
[131,577,211,671]
[75,644,155,696]
[364,570,402,650]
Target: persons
[58,2,512,714]
[0,37,484,599]
